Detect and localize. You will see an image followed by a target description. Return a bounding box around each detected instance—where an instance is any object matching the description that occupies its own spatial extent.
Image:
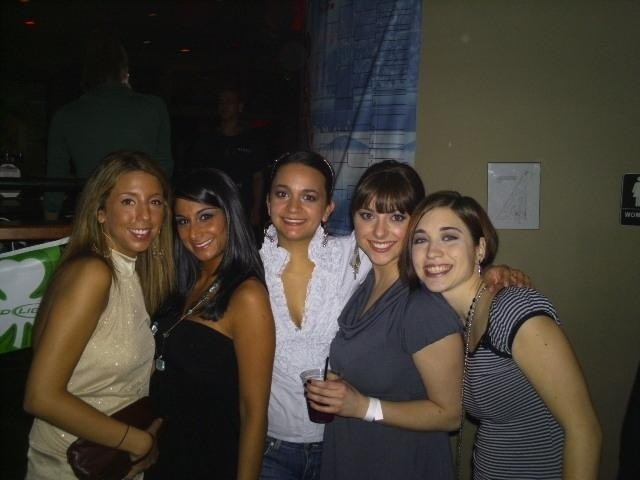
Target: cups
[300,368,344,424]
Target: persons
[304,157,465,480]
[197,87,265,227]
[258,148,533,480]
[44,41,174,220]
[144,165,276,478]
[610,365,640,480]
[398,189,603,479]
[22,149,176,480]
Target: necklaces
[151,276,221,372]
[455,281,489,474]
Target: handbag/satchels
[68,396,158,480]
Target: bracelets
[114,424,130,450]
[364,396,385,425]
